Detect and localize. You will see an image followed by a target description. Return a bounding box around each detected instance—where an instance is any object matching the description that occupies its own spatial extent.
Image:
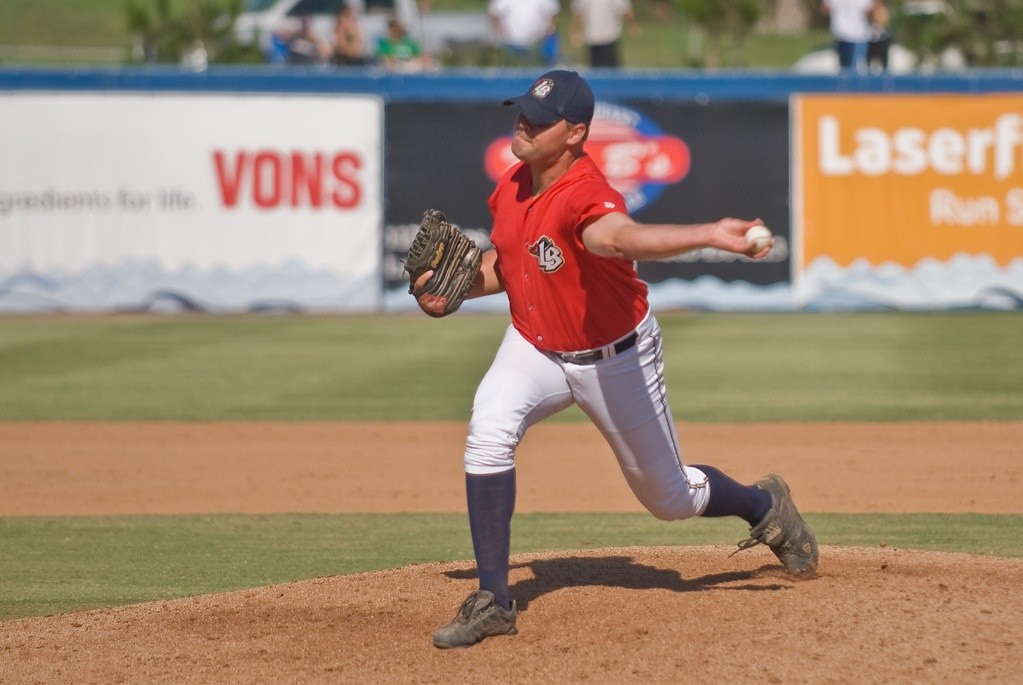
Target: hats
[503,69,596,127]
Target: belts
[554,329,639,366]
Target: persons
[283,0,436,79]
[823,1,897,76]
[403,70,820,650]
[485,1,643,70]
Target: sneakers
[738,472,820,579]
[433,589,519,649]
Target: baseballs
[744,225,773,252]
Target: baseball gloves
[406,207,483,319]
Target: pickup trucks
[221,1,494,71]
